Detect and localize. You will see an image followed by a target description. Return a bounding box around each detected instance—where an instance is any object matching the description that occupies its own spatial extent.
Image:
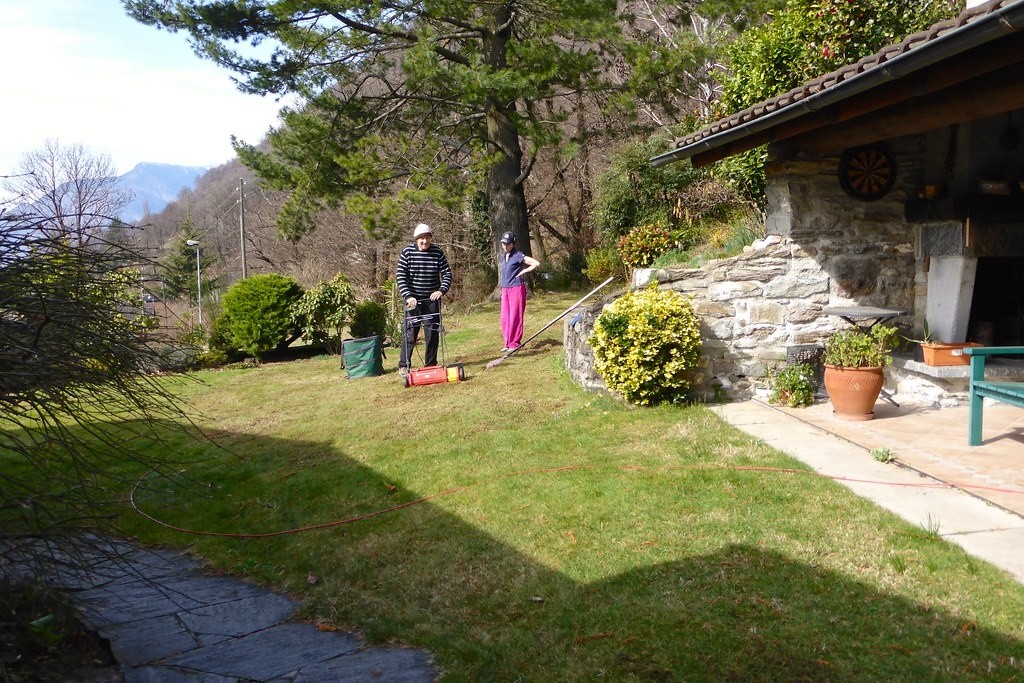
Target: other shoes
[507,348,518,353]
[399,367,407,374]
[500,347,508,352]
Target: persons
[397,222,451,378]
[498,231,540,353]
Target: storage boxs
[787,345,829,399]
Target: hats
[414,223,432,241]
[500,231,517,244]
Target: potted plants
[819,322,901,421]
[899,318,985,366]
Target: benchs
[962,345,1024,446]
